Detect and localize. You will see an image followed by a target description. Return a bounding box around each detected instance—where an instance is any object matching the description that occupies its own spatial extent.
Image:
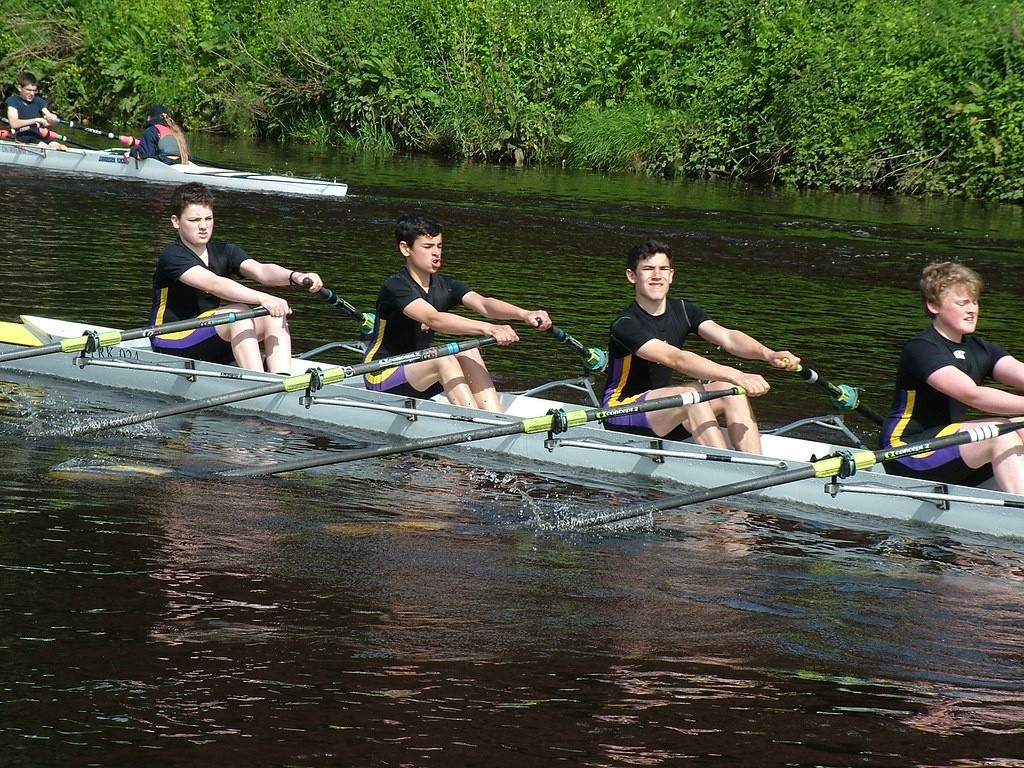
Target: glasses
[22,87,38,93]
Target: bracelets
[289,270,299,286]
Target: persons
[6,73,66,149]
[149,181,323,376]
[602,240,800,456]
[124,106,190,166]
[363,216,552,414]
[877,262,1024,496]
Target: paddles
[576,421,1024,530]
[782,357,887,424]
[535,316,611,378]
[37,337,497,438]
[224,385,747,478]
[40,129,104,150]
[303,277,379,334]
[0,307,274,365]
[41,117,140,148]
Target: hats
[146,105,167,117]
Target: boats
[0,136,348,199]
[0,314,1024,550]
